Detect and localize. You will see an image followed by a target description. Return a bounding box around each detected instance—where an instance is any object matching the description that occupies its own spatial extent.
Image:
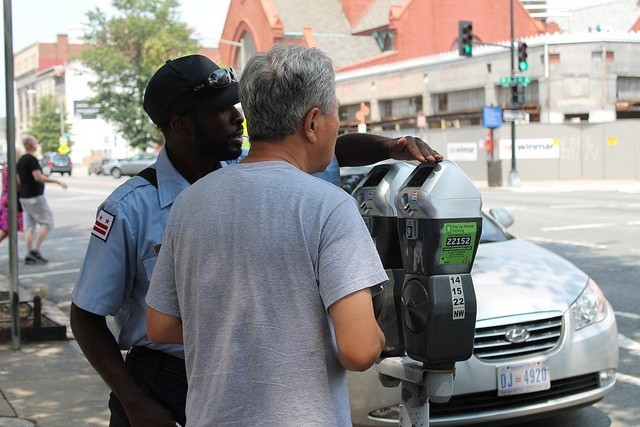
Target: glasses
[164,66,234,115]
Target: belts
[130,345,186,378]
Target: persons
[0,148,24,241]
[16,137,67,264]
[145,42,386,426]
[68,52,446,425]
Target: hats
[142,54,241,128]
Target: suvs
[48,155,72,175]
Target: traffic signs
[503,110,526,122]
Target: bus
[616,76,640,118]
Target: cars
[103,153,158,178]
[339,159,619,427]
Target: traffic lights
[518,42,528,71]
[459,22,472,56]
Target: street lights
[189,32,244,76]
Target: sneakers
[30,250,48,263]
[24,257,37,265]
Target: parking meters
[352,162,483,427]
